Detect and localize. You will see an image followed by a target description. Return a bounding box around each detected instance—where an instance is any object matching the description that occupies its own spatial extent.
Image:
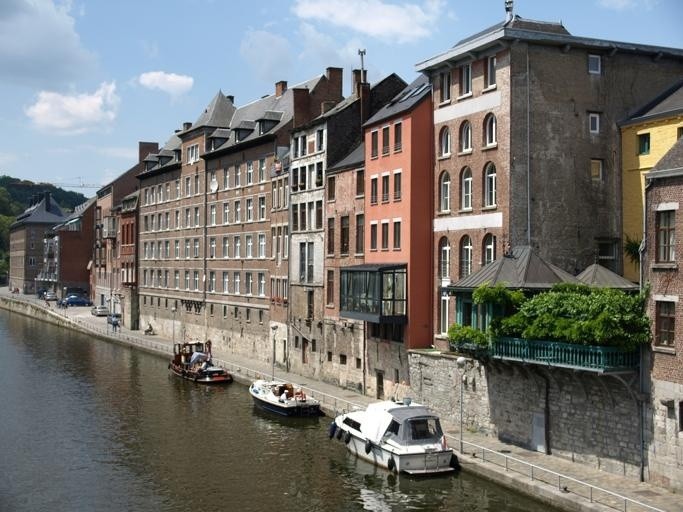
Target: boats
[329,397,456,475]
[248,379,320,416]
[169,339,233,384]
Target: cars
[44,291,93,306]
[91,306,121,325]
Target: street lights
[455,357,466,454]
[270,324,278,381]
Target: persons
[190,358,212,373]
[279,387,306,404]
[144,322,153,335]
[111,316,120,333]
